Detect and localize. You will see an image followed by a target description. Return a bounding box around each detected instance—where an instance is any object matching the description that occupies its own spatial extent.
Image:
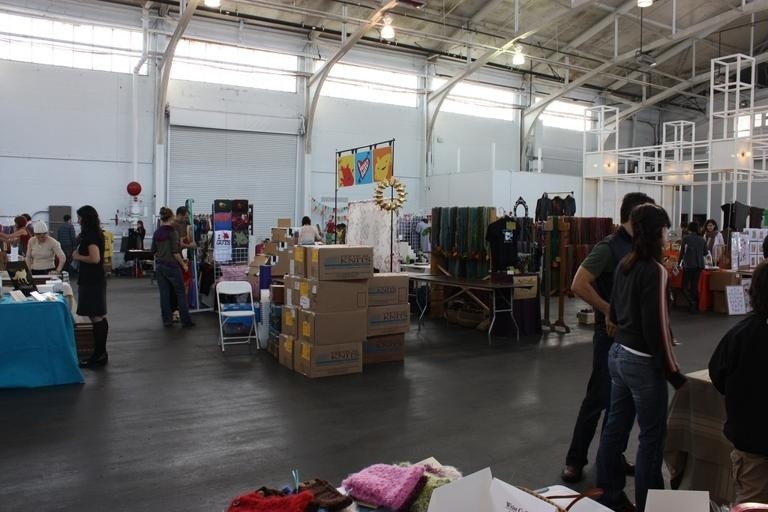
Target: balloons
[127,182,141,196]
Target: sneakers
[598,490,636,511]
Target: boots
[79,318,108,368]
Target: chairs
[216,279,262,350]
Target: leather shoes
[561,464,583,482]
[626,458,635,474]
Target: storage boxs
[264,243,411,377]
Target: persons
[172,206,194,251]
[24,221,66,274]
[708,260,767,503]
[299,216,322,245]
[596,203,690,511]
[1,216,30,255]
[136,220,146,250]
[678,221,708,315]
[705,221,725,267]
[560,193,657,482]
[151,207,197,328]
[414,218,432,253]
[70,205,109,368]
[57,214,74,272]
[22,212,33,234]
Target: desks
[398,271,532,345]
[0,277,85,387]
[665,265,742,315]
[124,248,154,278]
[665,366,734,504]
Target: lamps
[511,45,526,67]
[382,16,396,40]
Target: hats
[33,220,48,234]
